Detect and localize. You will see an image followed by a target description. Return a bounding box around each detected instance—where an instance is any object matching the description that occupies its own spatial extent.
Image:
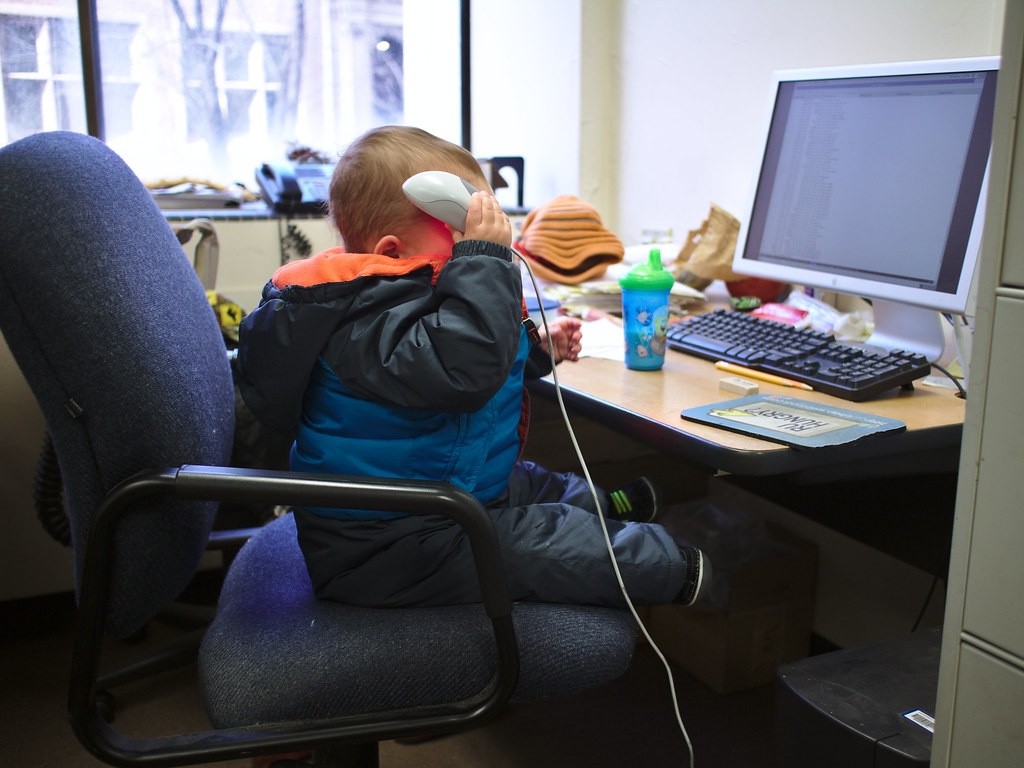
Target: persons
[242,126,762,616]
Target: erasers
[716,377,759,396]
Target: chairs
[0,131,647,768]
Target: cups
[619,248,674,372]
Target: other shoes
[674,544,711,607]
[609,476,658,523]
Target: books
[542,264,707,313]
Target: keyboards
[667,308,931,402]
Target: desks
[524,271,964,477]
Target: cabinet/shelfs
[929,0,1024,768]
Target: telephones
[253,161,334,215]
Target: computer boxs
[774,624,943,768]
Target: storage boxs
[566,463,820,698]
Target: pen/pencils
[715,361,815,392]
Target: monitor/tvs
[733,54,1001,365]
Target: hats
[520,192,625,268]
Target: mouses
[402,171,482,234]
